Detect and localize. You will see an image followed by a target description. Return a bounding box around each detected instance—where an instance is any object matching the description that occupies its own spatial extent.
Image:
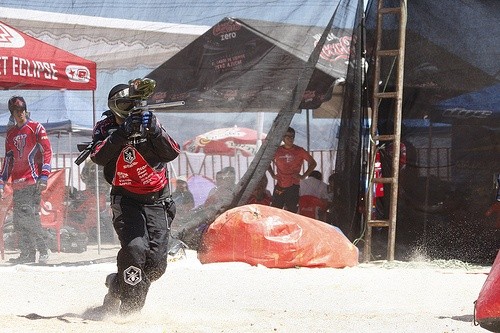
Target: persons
[80,160,110,195]
[172,167,272,216]
[0,96,53,265]
[267,127,317,212]
[90,84,180,314]
[299,171,335,201]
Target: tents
[0,20,101,254]
[423,82,500,247]
[143,16,369,155]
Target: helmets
[108,84,136,121]
[8,96,27,113]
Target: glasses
[285,135,294,139]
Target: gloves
[36,175,47,191]
[123,109,142,134]
[142,112,161,135]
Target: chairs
[297,194,329,220]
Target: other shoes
[9,251,35,263]
[39,249,52,263]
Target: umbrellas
[181,124,268,172]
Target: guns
[126,78,185,145]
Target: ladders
[362,0,407,261]
